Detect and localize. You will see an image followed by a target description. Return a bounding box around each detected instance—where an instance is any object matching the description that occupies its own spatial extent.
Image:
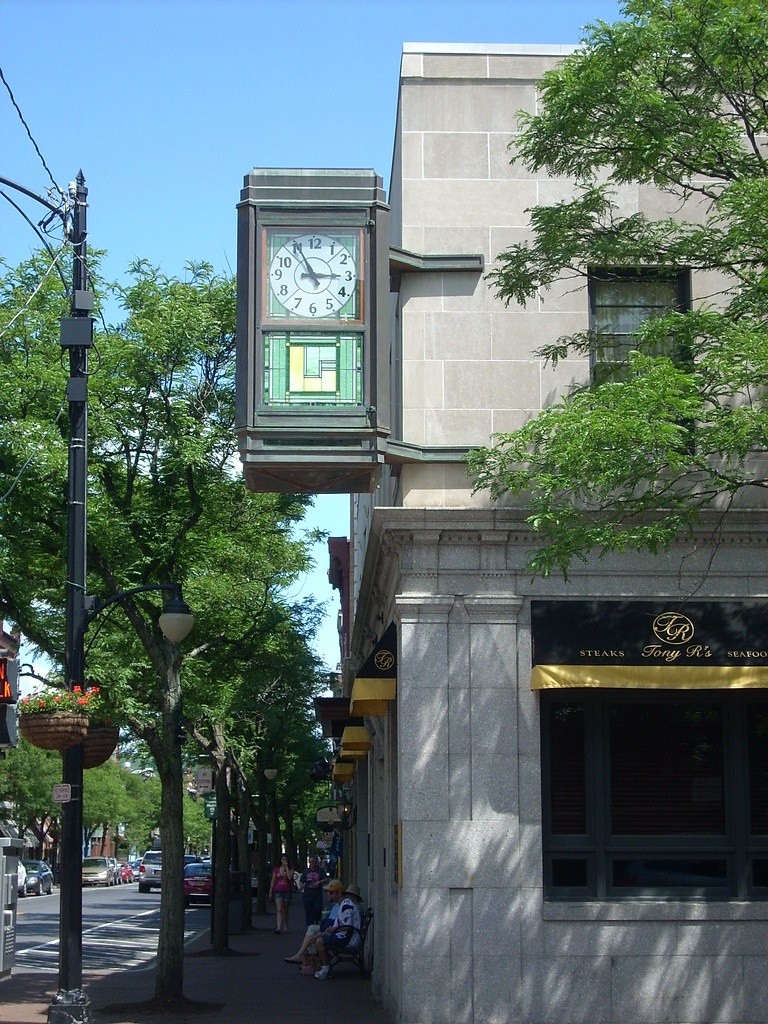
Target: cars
[182,862,213,908]
[14,858,27,896]
[137,850,163,892]
[108,856,122,885]
[118,861,134,883]
[21,860,54,897]
[183,853,202,870]
[128,861,139,881]
[81,856,114,887]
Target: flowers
[90,702,129,729]
[17,685,100,716]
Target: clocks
[269,234,357,319]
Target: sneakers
[314,965,334,981]
[283,954,303,964]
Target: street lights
[50,174,194,1022]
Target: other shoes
[274,927,290,934]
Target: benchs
[327,906,375,980]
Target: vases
[59,727,121,770]
[19,713,89,750]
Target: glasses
[327,892,335,896]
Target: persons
[283,884,364,965]
[311,880,361,980]
[300,854,329,932]
[268,854,294,934]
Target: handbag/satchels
[298,952,322,976]
[319,917,336,934]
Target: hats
[323,879,344,893]
[343,884,363,903]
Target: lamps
[336,794,357,830]
